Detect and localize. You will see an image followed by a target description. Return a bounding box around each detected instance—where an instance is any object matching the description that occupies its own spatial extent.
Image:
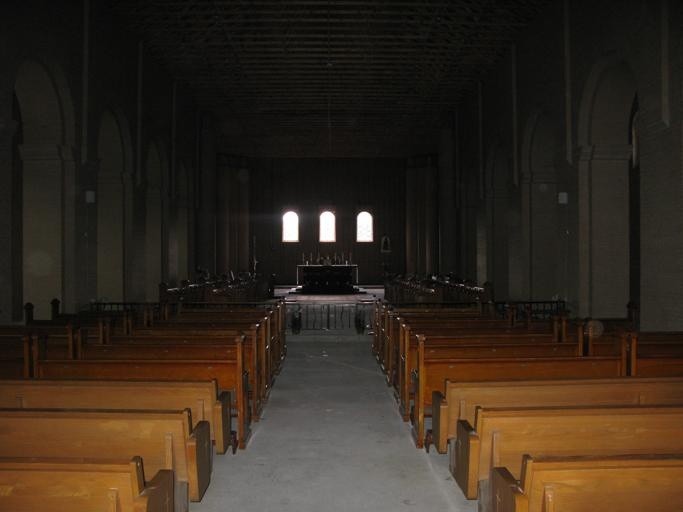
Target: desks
[296,264,359,288]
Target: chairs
[385,272,487,299]
[162,267,277,301]
